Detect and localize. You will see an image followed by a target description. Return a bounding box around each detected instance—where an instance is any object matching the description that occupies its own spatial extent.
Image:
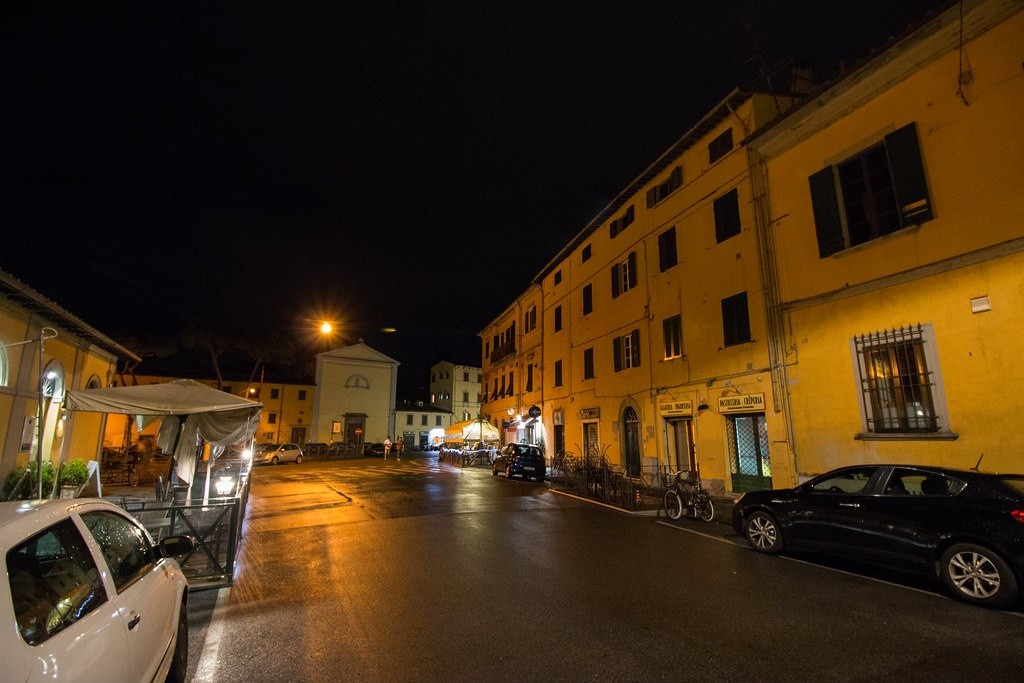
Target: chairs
[119,466,246,574]
[887,477,938,495]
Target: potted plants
[52,458,88,499]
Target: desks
[137,517,178,545]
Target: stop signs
[355,428,362,436]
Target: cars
[729,460,1024,610]
[0,497,192,683]
[366,442,386,456]
[492,443,546,482]
[253,442,304,465]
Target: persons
[384,436,404,462]
[439,442,503,464]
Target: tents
[444,419,501,442]
[63,379,263,592]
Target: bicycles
[663,469,715,522]
[100,455,140,487]
